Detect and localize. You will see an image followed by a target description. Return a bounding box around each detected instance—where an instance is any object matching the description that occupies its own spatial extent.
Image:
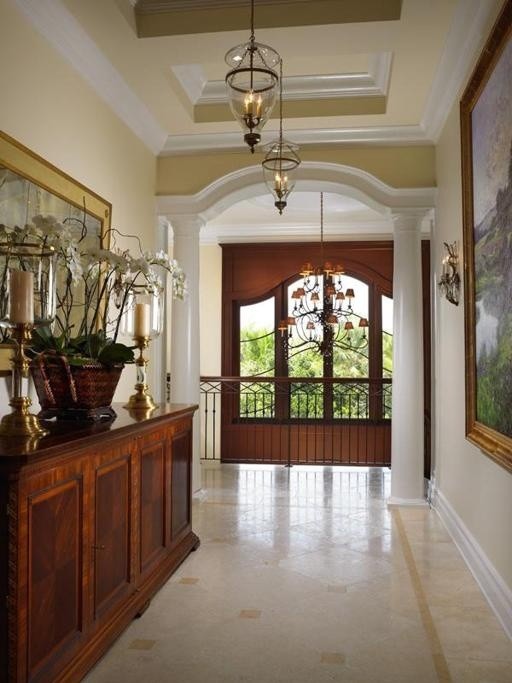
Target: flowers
[1,191,190,354]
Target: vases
[27,357,129,429]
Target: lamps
[225,1,282,155]
[436,238,463,307]
[278,191,371,362]
[262,83,301,214]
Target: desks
[0,399,204,682]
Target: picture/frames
[1,129,114,380]
[455,2,512,481]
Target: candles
[6,267,37,331]
[131,294,154,343]
